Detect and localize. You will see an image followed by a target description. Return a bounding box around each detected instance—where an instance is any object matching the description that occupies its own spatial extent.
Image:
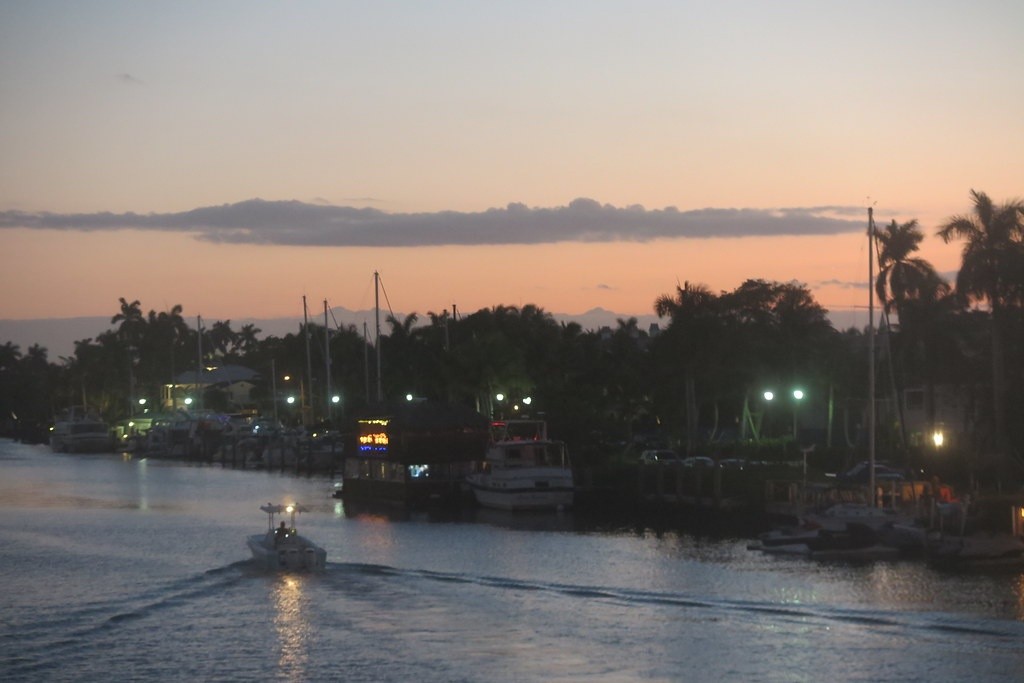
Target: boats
[804,205,1024,565]
[465,419,580,513]
[247,502,328,568]
[48,267,490,496]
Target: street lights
[793,388,806,440]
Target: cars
[640,450,803,481]
[839,461,904,493]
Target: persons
[274,522,290,548]
